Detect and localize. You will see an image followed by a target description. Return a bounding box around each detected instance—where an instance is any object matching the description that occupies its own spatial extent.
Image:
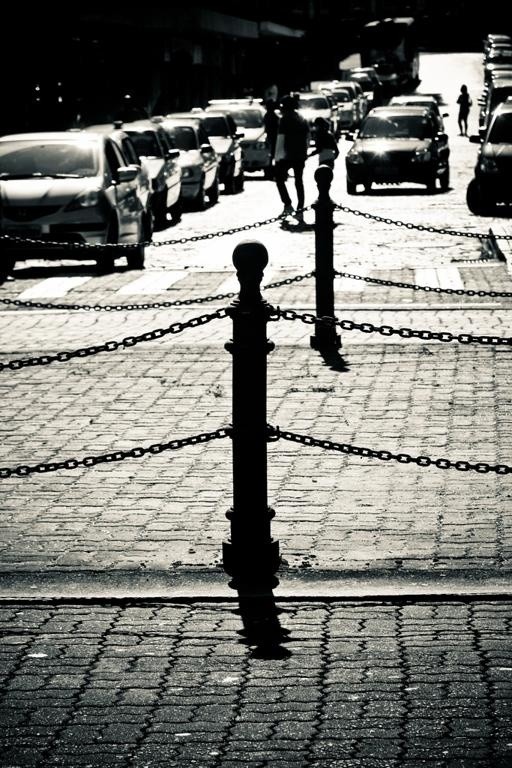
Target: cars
[0,130,153,283]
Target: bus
[358,14,424,95]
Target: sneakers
[277,204,294,218]
[294,210,307,225]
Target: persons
[457,85,472,137]
[264,99,278,145]
[271,94,309,222]
[308,116,339,170]
